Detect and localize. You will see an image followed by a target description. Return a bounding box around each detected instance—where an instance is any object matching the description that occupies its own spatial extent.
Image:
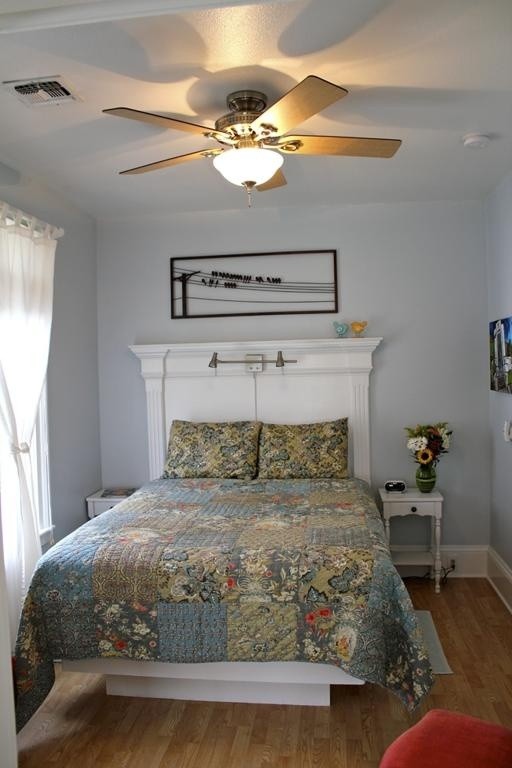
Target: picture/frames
[169,249,338,318]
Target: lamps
[208,350,297,368]
[212,142,285,192]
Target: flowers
[402,420,453,469]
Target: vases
[416,464,436,493]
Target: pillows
[160,417,349,478]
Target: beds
[15,477,434,734]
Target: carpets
[413,610,454,676]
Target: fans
[99,75,401,194]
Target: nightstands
[86,488,139,519]
[376,487,444,593]
